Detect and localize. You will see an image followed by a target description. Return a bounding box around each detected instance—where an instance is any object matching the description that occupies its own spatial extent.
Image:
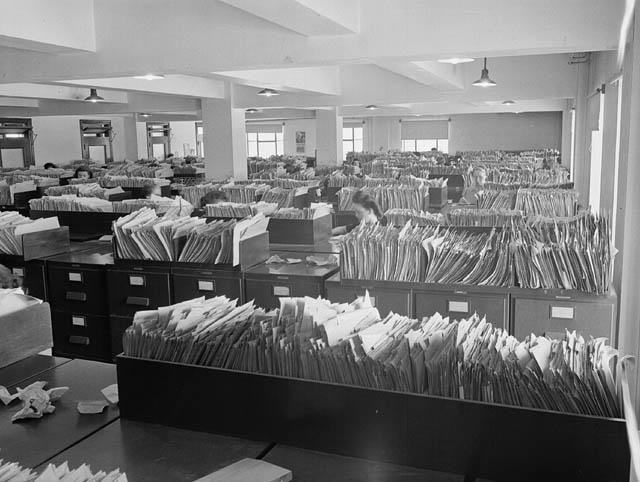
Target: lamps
[472,57,496,86]
[139,113,152,118]
[502,100,514,105]
[258,87,280,96]
[365,104,379,110]
[84,87,104,102]
[246,108,258,113]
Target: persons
[331,191,387,236]
[459,165,489,205]
[352,159,362,169]
[200,190,229,210]
[74,165,92,179]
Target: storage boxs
[245,274,321,311]
[107,316,138,362]
[170,268,243,308]
[49,308,108,358]
[411,292,510,333]
[1,256,45,301]
[106,265,170,316]
[512,295,617,347]
[323,282,411,319]
[46,259,106,316]
[116,350,626,480]
[0,156,615,256]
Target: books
[188,456,294,482]
